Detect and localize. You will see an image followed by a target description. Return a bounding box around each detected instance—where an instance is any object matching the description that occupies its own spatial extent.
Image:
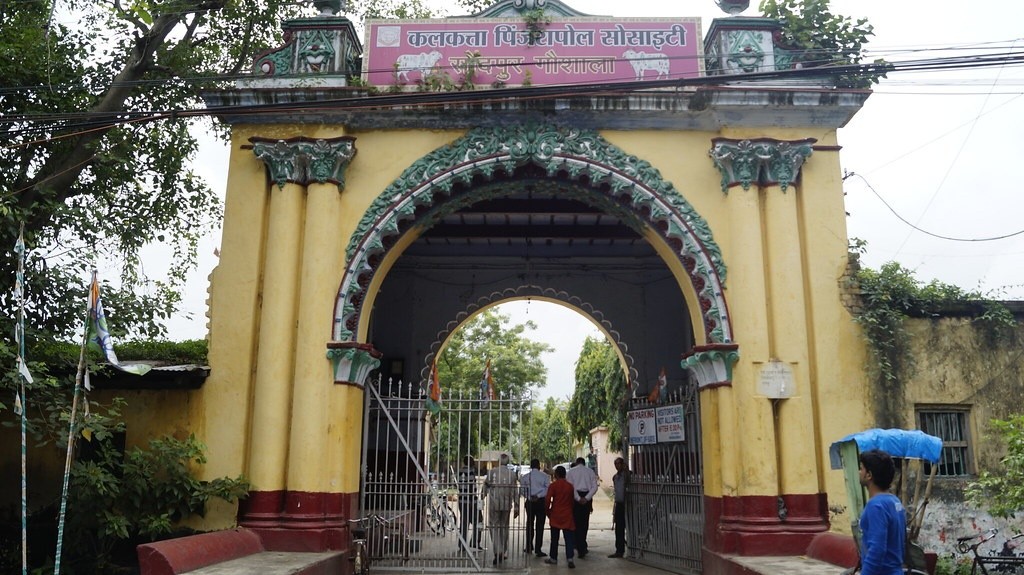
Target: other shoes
[537,552,547,557]
[524,547,534,552]
[493,553,507,564]
[608,553,622,558]
[569,562,575,568]
[472,545,487,550]
[578,553,584,559]
[545,558,558,564]
[459,546,466,551]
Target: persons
[566,456,599,559]
[857,449,907,575]
[544,466,577,569]
[480,454,520,564]
[586,446,600,486]
[520,459,551,558]
[457,455,484,553]
[607,457,633,559]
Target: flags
[86,273,154,379]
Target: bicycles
[956,527,1024,575]
[346,513,385,575]
[424,479,456,538]
